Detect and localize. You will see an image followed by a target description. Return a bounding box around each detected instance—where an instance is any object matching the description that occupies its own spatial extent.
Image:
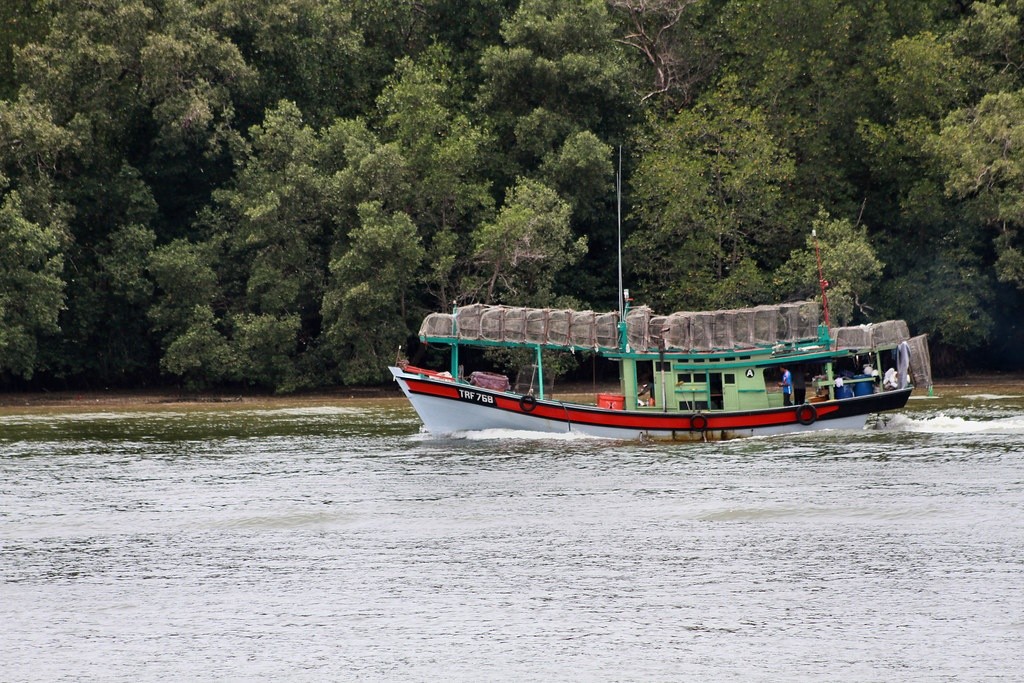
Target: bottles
[646,399,649,406]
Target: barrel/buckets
[836,369,855,399]
[855,374,873,397]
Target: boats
[387,144,934,444]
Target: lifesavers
[795,404,817,426]
[689,415,708,431]
[519,395,536,412]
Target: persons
[791,362,810,405]
[638,377,655,407]
[777,364,793,406]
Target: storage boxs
[597,393,626,410]
